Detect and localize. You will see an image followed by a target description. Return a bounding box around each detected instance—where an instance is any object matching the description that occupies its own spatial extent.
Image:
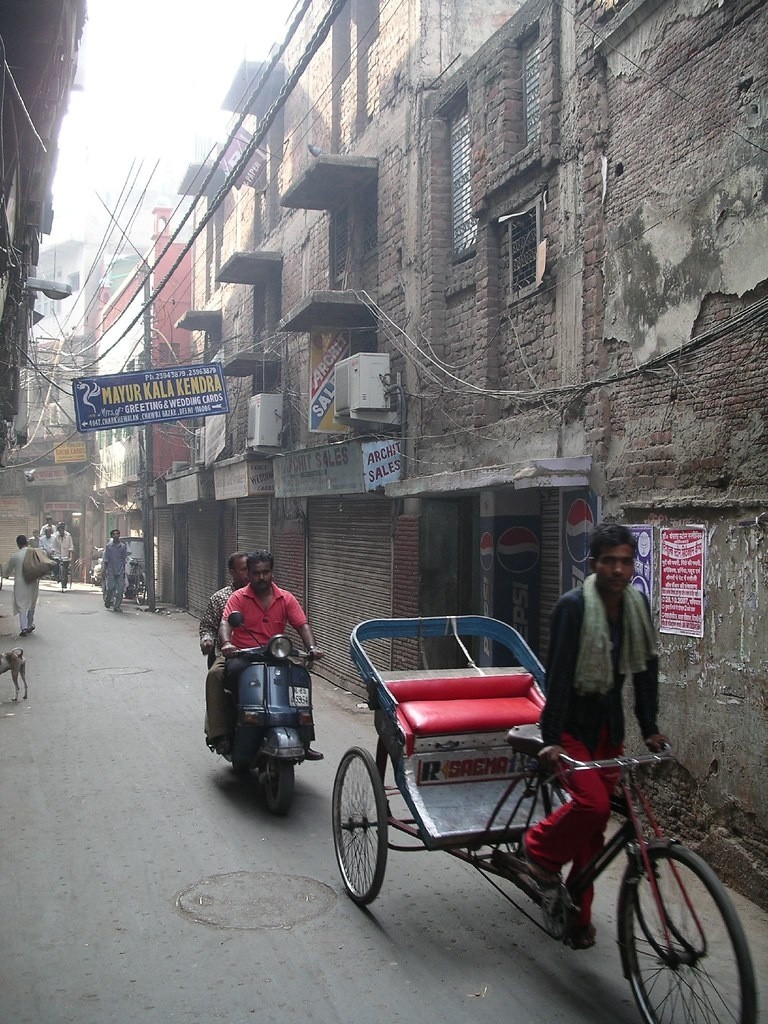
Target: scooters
[204,609,324,818]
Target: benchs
[384,671,551,757]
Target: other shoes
[113,608,123,613]
[62,582,67,588]
[305,748,324,761]
[216,738,229,754]
[105,602,110,608]
[568,919,596,949]
[522,833,549,884]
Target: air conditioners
[335,352,390,417]
[195,428,204,465]
[247,393,284,448]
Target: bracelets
[222,640,230,647]
[308,645,318,650]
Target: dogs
[0,647,29,702]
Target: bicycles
[128,560,147,606]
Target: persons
[101,529,127,612]
[39,527,56,556]
[53,525,73,588]
[28,528,40,548]
[40,515,56,535]
[199,552,248,755]
[5,535,39,636]
[56,521,70,533]
[219,549,324,761]
[522,524,672,951]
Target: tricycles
[331,613,767,1018]
[53,559,72,593]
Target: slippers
[19,629,27,637]
[27,624,35,633]
